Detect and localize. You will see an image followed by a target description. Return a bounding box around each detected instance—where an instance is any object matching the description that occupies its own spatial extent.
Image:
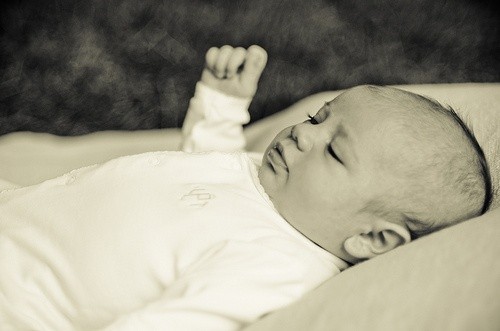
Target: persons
[0,44,495,331]
